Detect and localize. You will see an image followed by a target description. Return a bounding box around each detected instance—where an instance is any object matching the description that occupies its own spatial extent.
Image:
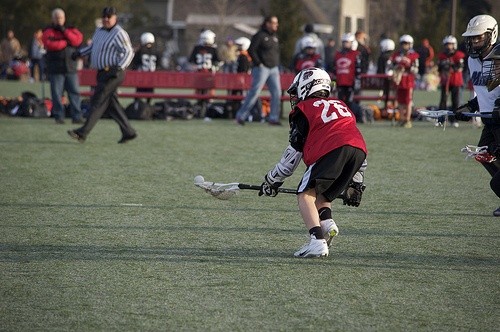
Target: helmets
[442,35,458,52]
[462,14,498,59]
[342,32,356,42]
[287,67,331,109]
[140,32,155,45]
[380,39,395,52]
[235,37,251,50]
[400,34,414,49]
[300,37,316,49]
[200,29,216,45]
[481,44,499,92]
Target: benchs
[75,69,398,118]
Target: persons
[385,34,419,129]
[233,36,251,96]
[0,30,31,81]
[67,5,138,144]
[454,15,500,177]
[291,24,395,109]
[258,67,367,258]
[28,29,46,83]
[41,8,87,124]
[236,15,282,125]
[221,38,241,95]
[83,38,93,69]
[416,38,434,80]
[486,44,500,216]
[128,32,158,105]
[188,30,224,105]
[434,35,464,128]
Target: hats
[304,24,313,32]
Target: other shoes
[73,118,86,123]
[56,118,65,124]
[398,120,413,128]
[118,134,137,143]
[68,130,85,144]
[269,120,282,125]
[237,116,244,125]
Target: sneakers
[453,122,459,127]
[293,234,329,258]
[320,218,339,247]
[493,206,500,217]
[435,122,444,127]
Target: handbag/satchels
[7,91,234,119]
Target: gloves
[491,98,500,122]
[454,102,475,121]
[258,171,284,198]
[111,67,121,78]
[72,51,80,60]
[343,177,366,207]
[57,24,65,32]
